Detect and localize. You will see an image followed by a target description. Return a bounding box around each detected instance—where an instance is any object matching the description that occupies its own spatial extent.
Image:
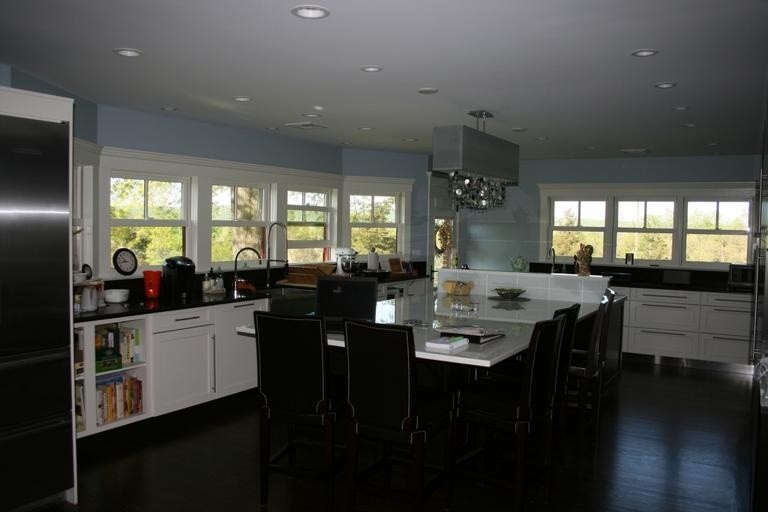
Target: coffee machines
[335,247,357,277]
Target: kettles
[81,286,98,312]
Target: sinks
[256,288,313,297]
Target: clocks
[112,247,139,277]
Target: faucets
[267,222,288,288]
[233,247,260,300]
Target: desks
[235,291,628,395]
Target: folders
[441,332,506,344]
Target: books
[433,325,506,336]
[73,320,144,435]
[424,337,470,352]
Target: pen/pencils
[447,336,464,343]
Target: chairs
[457,287,618,483]
[252,310,350,507]
[342,318,446,512]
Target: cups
[143,271,161,299]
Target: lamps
[431,110,522,212]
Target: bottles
[201,266,224,294]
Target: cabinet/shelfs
[609,289,698,368]
[702,293,754,364]
[72,298,274,441]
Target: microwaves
[726,263,764,292]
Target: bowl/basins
[491,301,525,311]
[103,288,129,303]
[494,288,525,299]
[72,273,87,282]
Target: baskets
[444,280,474,296]
